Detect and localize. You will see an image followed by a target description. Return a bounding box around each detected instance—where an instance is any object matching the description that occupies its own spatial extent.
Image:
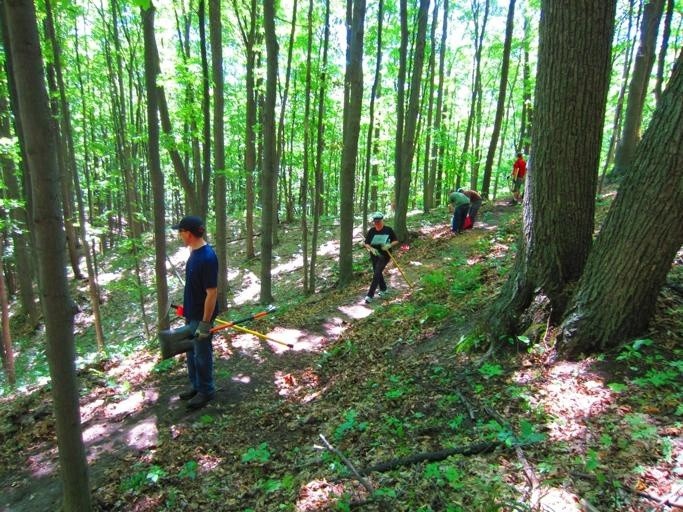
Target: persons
[446,189,471,234]
[507,152,527,206]
[169,213,220,409]
[359,211,399,303]
[453,187,483,228]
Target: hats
[172,215,203,237]
[372,212,383,221]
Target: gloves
[370,247,380,256]
[195,321,211,340]
[381,244,391,251]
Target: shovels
[158,309,276,361]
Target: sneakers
[365,296,372,303]
[186,391,217,408]
[178,388,197,399]
[378,290,388,295]
